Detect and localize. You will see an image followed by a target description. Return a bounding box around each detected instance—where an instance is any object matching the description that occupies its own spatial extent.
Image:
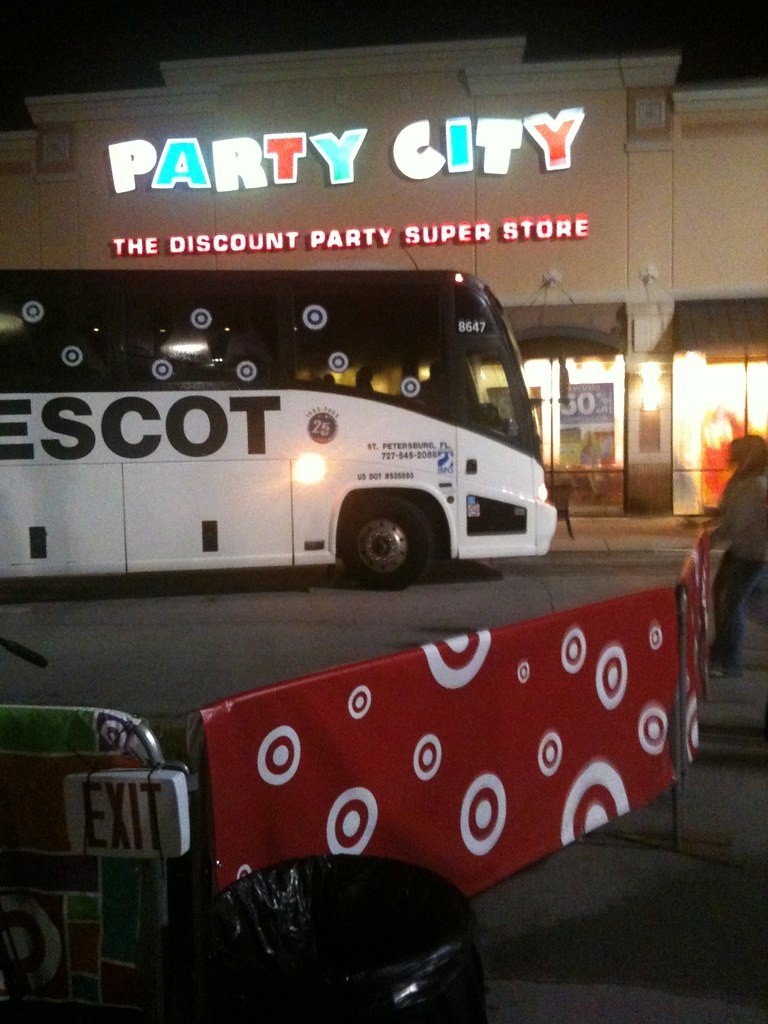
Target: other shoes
[709,667,740,678]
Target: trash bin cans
[202,854,487,1024]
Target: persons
[478,402,498,417]
[702,435,768,677]
[354,370,373,391]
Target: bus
[0,269,559,591]
